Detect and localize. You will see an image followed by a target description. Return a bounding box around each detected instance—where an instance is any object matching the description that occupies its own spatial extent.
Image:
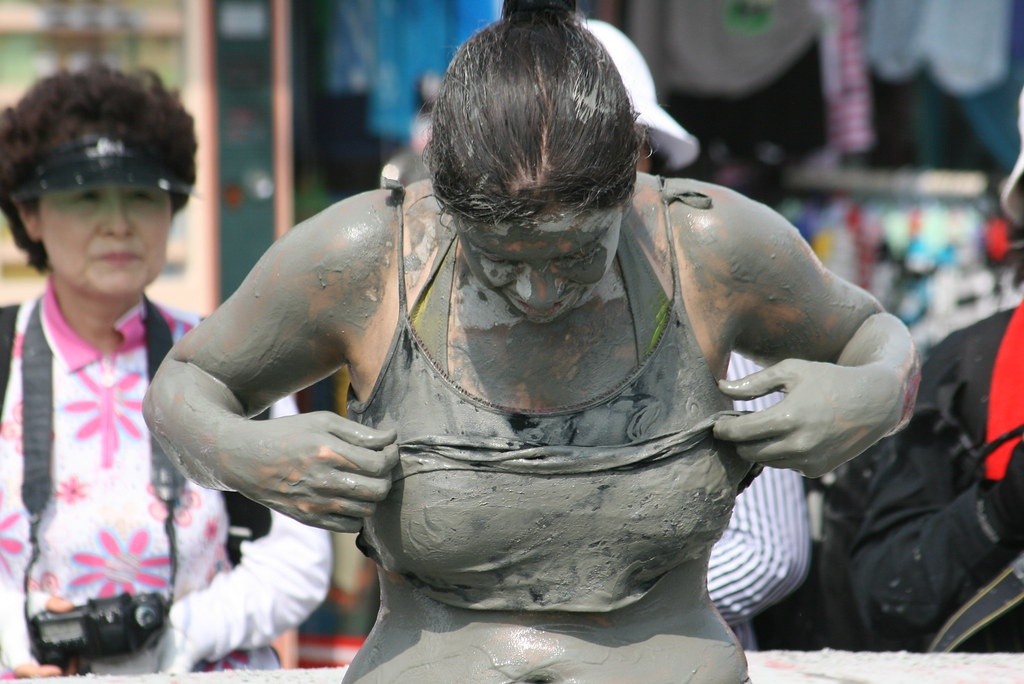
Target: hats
[574,17,700,173]
[5,130,196,203]
[1000,85,1024,228]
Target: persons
[140,0,922,684]
[0,20,1024,683]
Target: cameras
[29,590,166,678]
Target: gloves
[983,441,1024,552]
